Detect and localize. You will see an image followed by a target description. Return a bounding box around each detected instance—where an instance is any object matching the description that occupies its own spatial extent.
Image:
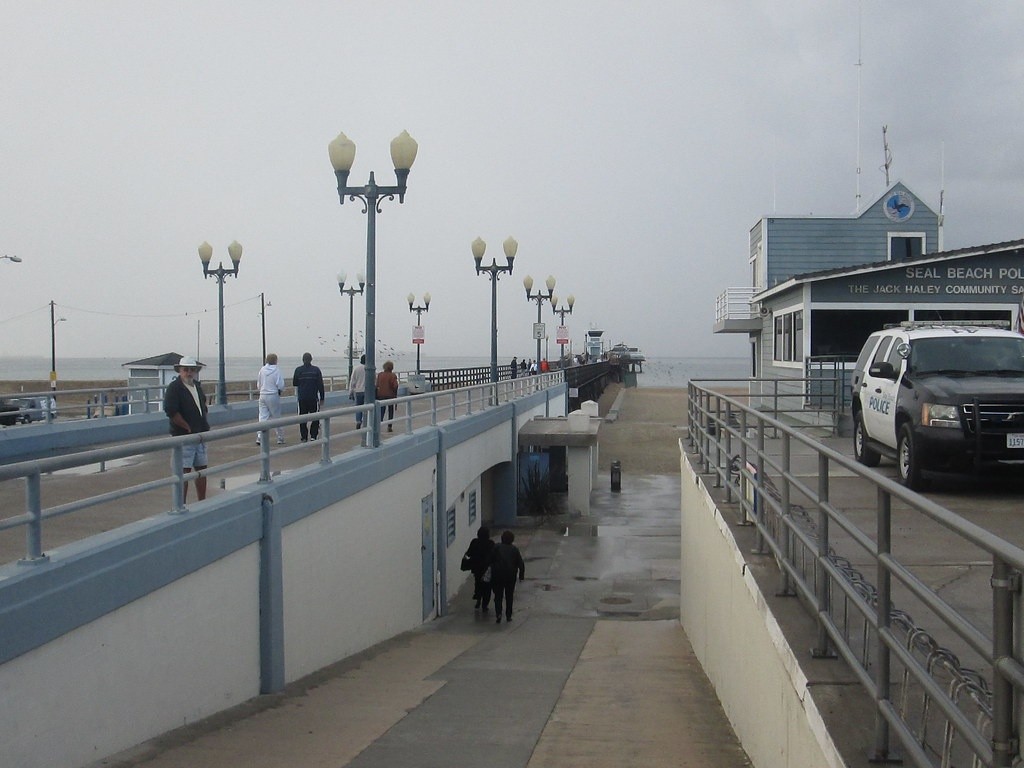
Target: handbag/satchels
[461,554,470,571]
[531,367,534,371]
[481,567,491,582]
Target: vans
[18,397,58,425]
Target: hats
[174,356,202,373]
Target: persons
[520,359,527,377]
[163,356,210,504]
[255,354,285,444]
[467,527,494,612]
[293,353,324,443]
[557,353,586,369]
[528,359,537,375]
[487,530,525,623]
[511,357,517,379]
[542,359,548,373]
[376,362,398,432]
[349,355,365,429]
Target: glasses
[184,368,195,372]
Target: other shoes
[507,617,512,621]
[256,437,261,444]
[496,615,501,623]
[475,601,481,609]
[277,437,286,444]
[388,427,392,432]
[482,608,488,612]
[356,423,361,429]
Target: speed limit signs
[533,323,545,339]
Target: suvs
[850,320,1024,492]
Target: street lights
[524,273,556,391]
[550,293,575,370]
[50,300,66,401]
[406,292,431,374]
[470,235,518,406]
[328,129,418,448]
[198,240,242,405]
[337,269,365,380]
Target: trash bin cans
[611,461,621,492]
[407,375,426,394]
